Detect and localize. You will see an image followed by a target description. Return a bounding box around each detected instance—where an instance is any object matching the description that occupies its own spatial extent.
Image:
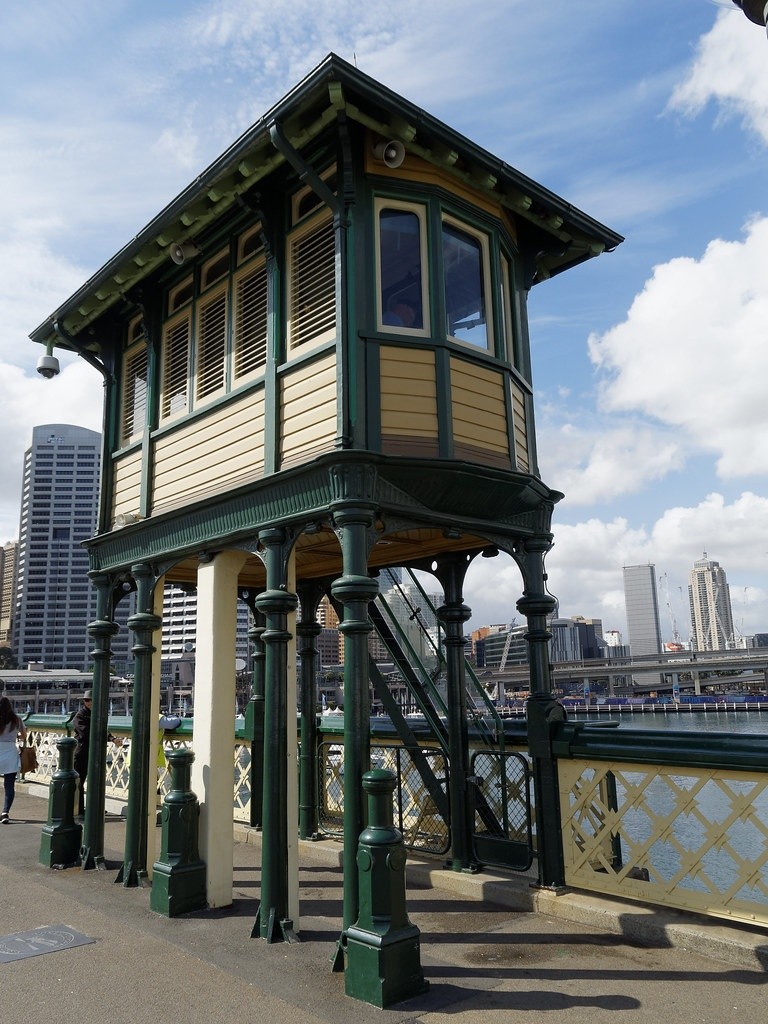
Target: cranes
[490,617,516,699]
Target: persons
[0,697,27,824]
[72,690,122,817]
[158,695,182,796]
[382,298,421,328]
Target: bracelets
[112,738,116,742]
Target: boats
[326,745,387,776]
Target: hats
[77,690,93,699]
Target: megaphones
[372,140,405,168]
[168,242,204,265]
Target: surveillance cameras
[36,356,60,379]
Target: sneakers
[0,812,9,823]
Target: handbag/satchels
[19,734,39,773]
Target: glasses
[83,699,93,702]
